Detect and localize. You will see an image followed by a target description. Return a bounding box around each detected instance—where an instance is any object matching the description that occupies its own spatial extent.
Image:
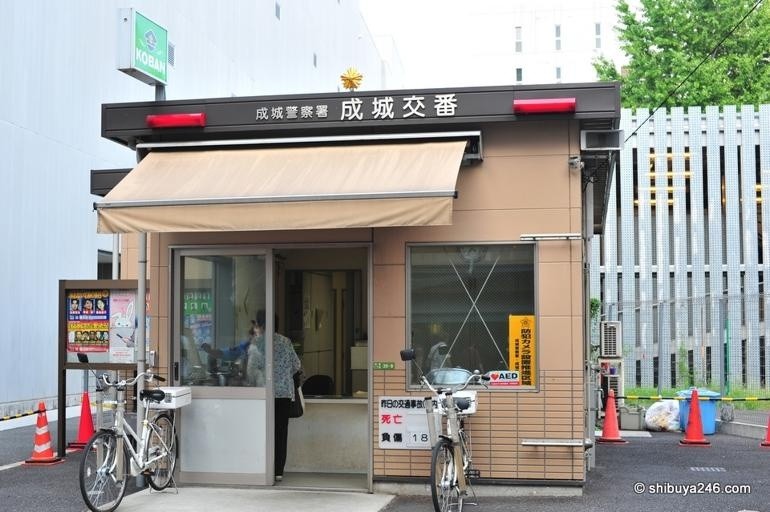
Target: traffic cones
[25,392,94,463]
[598,389,770,447]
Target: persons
[83,299,92,310]
[201,327,257,375]
[71,299,80,311]
[122,330,135,348]
[96,300,107,310]
[247,310,302,483]
[75,331,109,345]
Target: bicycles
[192,359,244,385]
[398,350,490,512]
[77,352,179,512]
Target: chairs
[301,374,336,396]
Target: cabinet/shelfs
[285,269,336,393]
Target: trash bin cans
[676,386,721,434]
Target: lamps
[146,113,207,128]
[513,97,576,115]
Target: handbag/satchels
[289,378,305,419]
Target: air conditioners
[597,320,624,418]
